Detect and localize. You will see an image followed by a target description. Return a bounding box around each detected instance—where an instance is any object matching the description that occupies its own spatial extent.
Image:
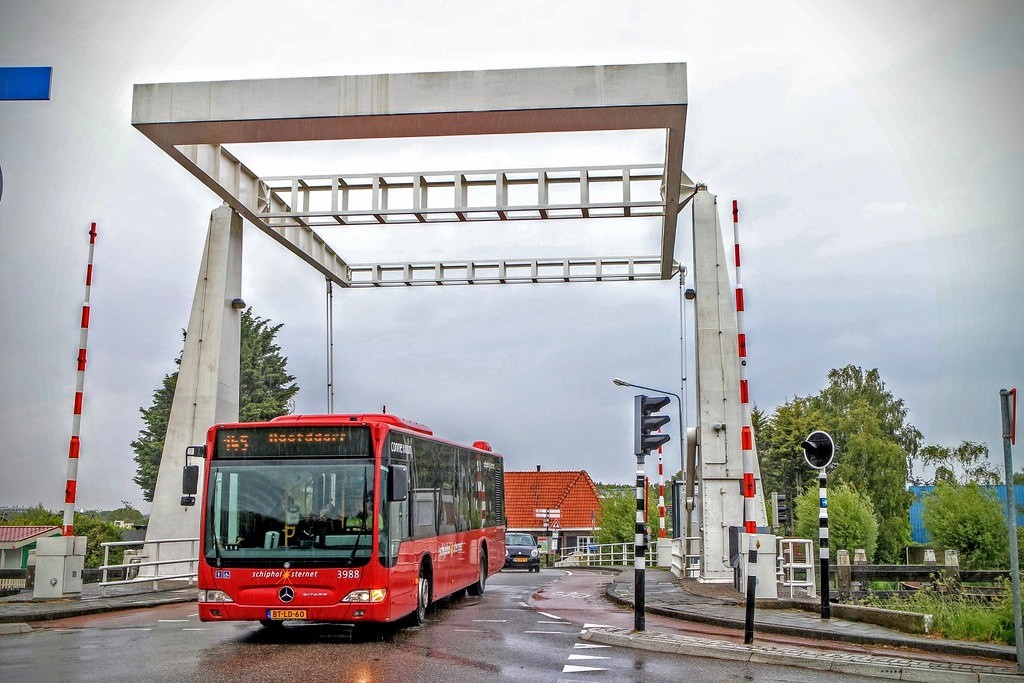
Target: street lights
[612,377,686,481]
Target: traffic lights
[800,439,828,460]
[777,494,788,523]
[633,394,671,455]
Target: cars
[500,532,541,572]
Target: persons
[273,496,309,552]
[342,495,385,537]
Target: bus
[177,414,507,630]
